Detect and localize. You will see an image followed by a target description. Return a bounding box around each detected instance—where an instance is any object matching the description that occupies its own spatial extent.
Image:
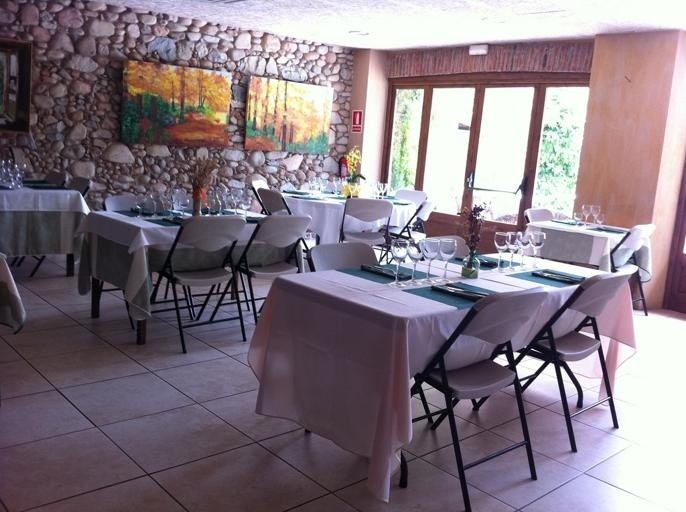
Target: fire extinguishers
[338,151,350,179]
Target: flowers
[345,145,366,182]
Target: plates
[596,225,625,234]
[361,262,414,280]
[530,269,585,285]
[280,188,311,195]
[128,206,266,226]
[431,282,486,302]
[553,218,586,226]
[454,254,503,267]
[21,179,69,190]
[292,195,321,200]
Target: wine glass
[387,236,457,288]
[203,186,253,219]
[305,174,388,200]
[574,201,605,228]
[134,186,189,221]
[0,157,25,191]
[491,230,545,273]
